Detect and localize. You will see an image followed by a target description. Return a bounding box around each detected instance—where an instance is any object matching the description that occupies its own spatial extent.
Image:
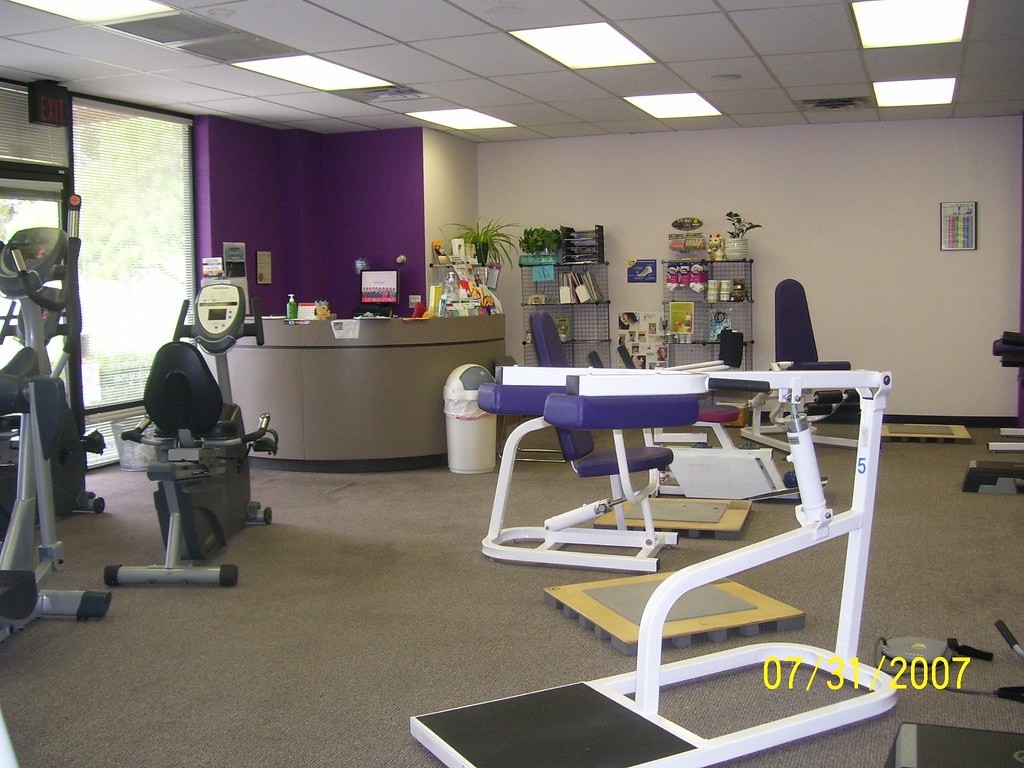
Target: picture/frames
[360,268,399,305]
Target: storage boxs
[560,286,572,304]
[717,399,748,428]
[451,238,466,264]
[576,285,591,303]
[464,243,478,264]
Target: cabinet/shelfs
[519,261,613,369]
[431,263,459,285]
[659,259,755,405]
[561,224,605,262]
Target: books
[558,270,603,303]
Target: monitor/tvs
[360,268,400,317]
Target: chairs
[769,278,852,424]
[495,356,567,464]
[529,311,676,532]
[143,340,240,449]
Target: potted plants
[434,215,521,272]
[725,211,764,260]
[517,227,562,254]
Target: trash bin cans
[440,363,501,475]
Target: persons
[618,312,667,369]
[362,287,396,302]
